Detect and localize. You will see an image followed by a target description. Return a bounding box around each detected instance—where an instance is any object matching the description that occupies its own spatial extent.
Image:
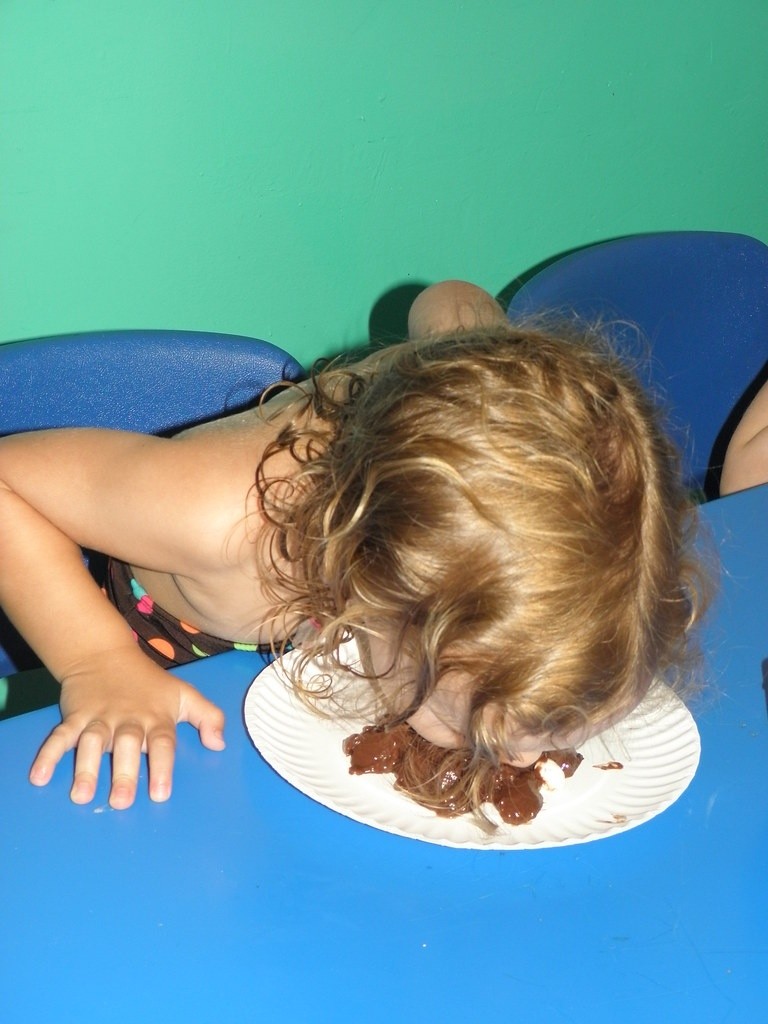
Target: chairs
[501,229,767,509]
[0,329,312,724]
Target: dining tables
[0,479,768,1024]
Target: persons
[719,377,767,497]
[1,279,693,812]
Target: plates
[241,638,707,853]
